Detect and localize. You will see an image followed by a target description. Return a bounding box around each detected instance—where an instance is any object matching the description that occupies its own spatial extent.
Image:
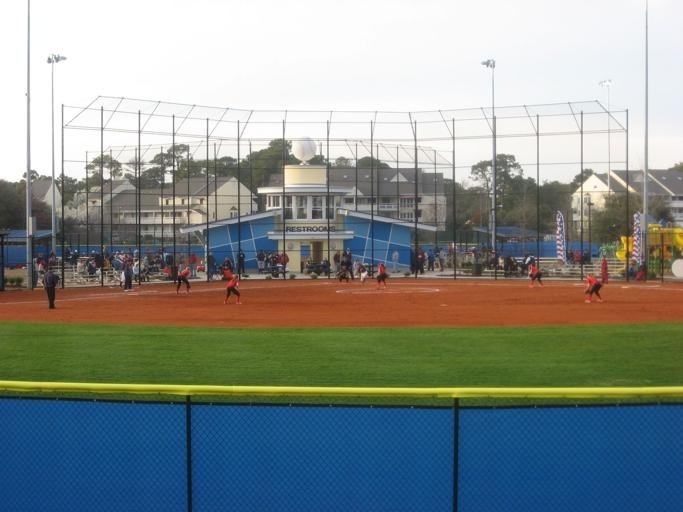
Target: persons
[34,243,647,309]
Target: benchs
[484,256,636,279]
[34,256,164,284]
